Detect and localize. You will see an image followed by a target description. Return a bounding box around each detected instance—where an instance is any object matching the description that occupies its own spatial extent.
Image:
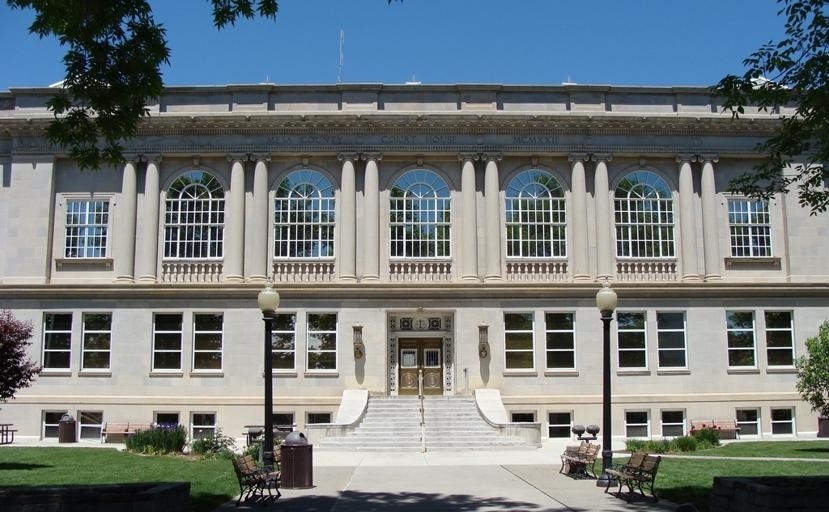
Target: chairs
[605,452,660,505]
[233,455,282,508]
[560,440,601,480]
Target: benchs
[101,422,153,447]
[690,419,741,440]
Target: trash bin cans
[278,431,317,490]
[57,414,78,443]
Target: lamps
[352,320,364,359]
[478,320,489,359]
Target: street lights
[597,276,619,489]
[258,277,280,472]
